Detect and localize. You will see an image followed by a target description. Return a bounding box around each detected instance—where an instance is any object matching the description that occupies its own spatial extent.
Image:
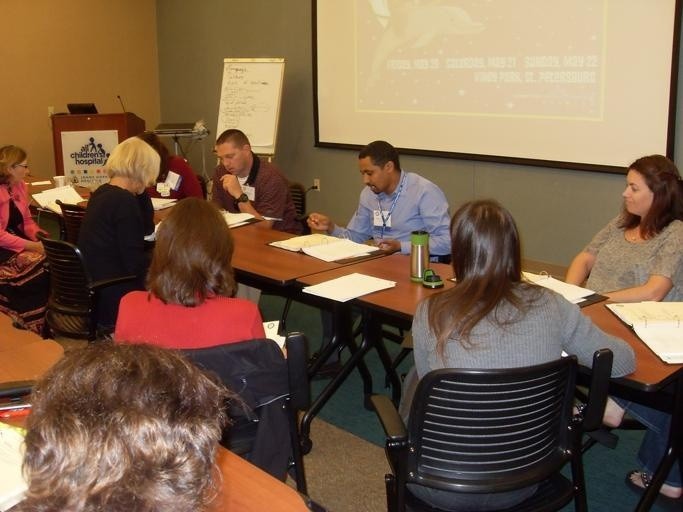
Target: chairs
[36,230,134,344]
[55,198,88,240]
[280,183,309,334]
[369,348,613,512]
[181,332,312,497]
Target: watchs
[234,193,248,204]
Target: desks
[1,305,63,391]
[295,253,683,455]
[31,185,392,380]
[1,394,311,512]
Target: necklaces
[626,227,645,242]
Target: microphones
[117,95,125,112]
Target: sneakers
[308,360,342,380]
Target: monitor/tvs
[67,103,98,114]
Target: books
[150,197,178,210]
[447,271,611,308]
[262,321,286,350]
[30,185,84,215]
[218,209,261,228]
[604,302,683,364]
[264,232,384,264]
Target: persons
[307,141,453,410]
[399,199,636,511]
[136,133,205,199]
[567,396,683,511]
[565,154,683,303]
[0,146,50,304]
[5,341,253,510]
[113,198,288,360]
[75,137,162,326]
[208,129,304,235]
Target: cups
[53,175,65,188]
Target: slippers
[626,469,682,509]
[574,403,620,449]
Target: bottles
[410,230,430,283]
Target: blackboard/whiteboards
[212,57,288,158]
